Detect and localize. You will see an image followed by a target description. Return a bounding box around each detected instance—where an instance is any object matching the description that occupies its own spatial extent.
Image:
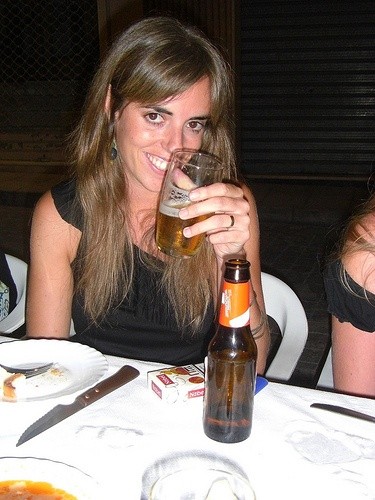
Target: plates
[0,457,104,500]
[0,339,109,402]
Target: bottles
[202,258,258,443]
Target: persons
[322,192,375,397]
[26,18,271,376]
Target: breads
[1,372,27,399]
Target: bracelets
[249,291,267,339]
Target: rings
[227,213,235,229]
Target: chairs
[0,253,27,334]
[257,273,308,384]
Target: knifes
[310,402,375,422]
[15,365,140,448]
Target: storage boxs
[147,362,206,403]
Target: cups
[154,150,224,260]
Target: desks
[0,334,375,500]
